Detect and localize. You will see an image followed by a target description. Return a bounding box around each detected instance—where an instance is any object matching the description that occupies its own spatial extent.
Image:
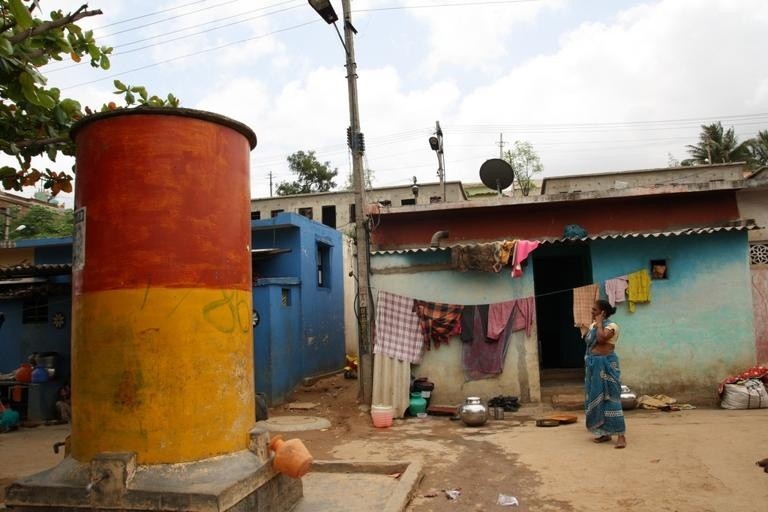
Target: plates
[449,416,460,421]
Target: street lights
[427,134,447,204]
[307,0,374,408]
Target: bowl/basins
[535,419,560,426]
[416,413,427,419]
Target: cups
[493,406,504,420]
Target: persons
[584,300,627,448]
[54,372,71,420]
